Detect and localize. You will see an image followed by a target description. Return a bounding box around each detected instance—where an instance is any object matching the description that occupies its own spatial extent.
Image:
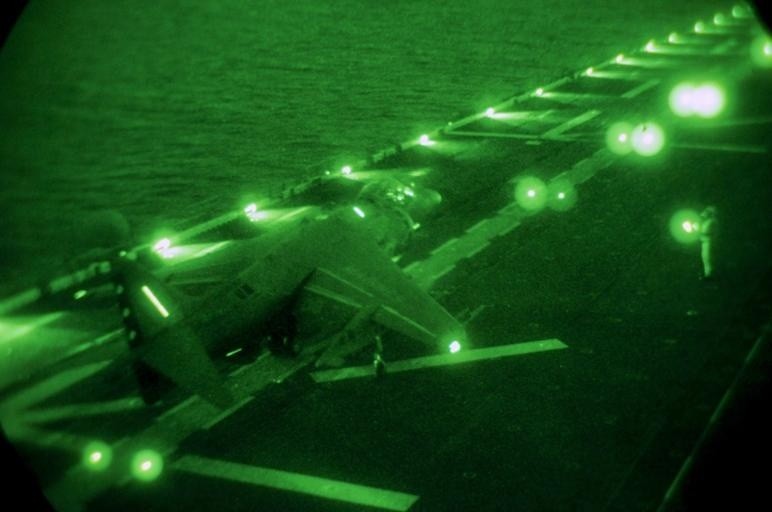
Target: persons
[698,207,720,281]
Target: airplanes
[109,171,474,410]
